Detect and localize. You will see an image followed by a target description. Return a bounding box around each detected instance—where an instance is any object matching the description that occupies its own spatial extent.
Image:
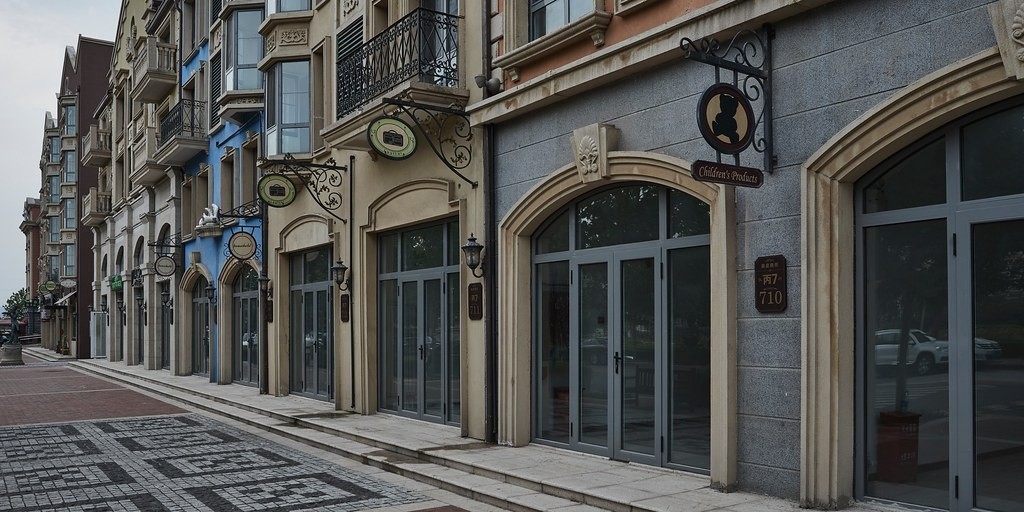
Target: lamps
[160,289,173,307]
[135,294,147,310]
[461,232,486,278]
[101,301,109,314]
[88,304,95,315]
[331,257,349,292]
[258,270,273,298]
[205,281,217,304]
[116,297,126,312]
[473,75,507,95]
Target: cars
[560,338,607,364]
[203,317,461,376]
[927,329,1002,366]
[873,329,942,377]
